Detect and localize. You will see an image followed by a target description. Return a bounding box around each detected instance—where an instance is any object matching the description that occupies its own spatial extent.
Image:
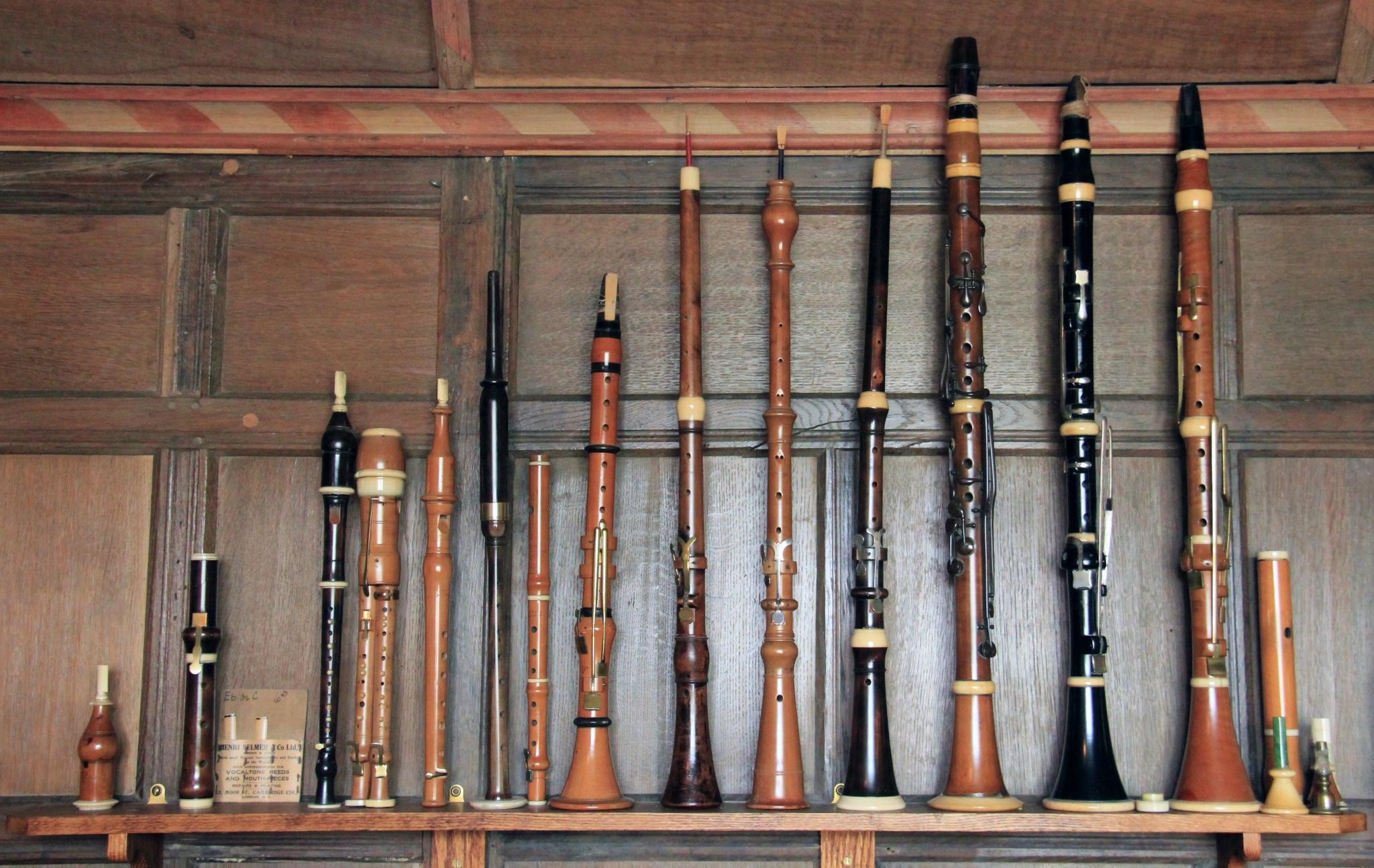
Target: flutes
[1258,548,1305,798]
[927,35,1026,814]
[522,452,552,806]
[307,368,360,811]
[553,270,626,811]
[745,126,807,811]
[343,427,408,809]
[73,665,119,810]
[421,378,458,809]
[830,105,907,812]
[1040,76,1138,817]
[176,553,222,808]
[661,107,723,812]
[468,269,526,811]
[1165,77,1258,815]
